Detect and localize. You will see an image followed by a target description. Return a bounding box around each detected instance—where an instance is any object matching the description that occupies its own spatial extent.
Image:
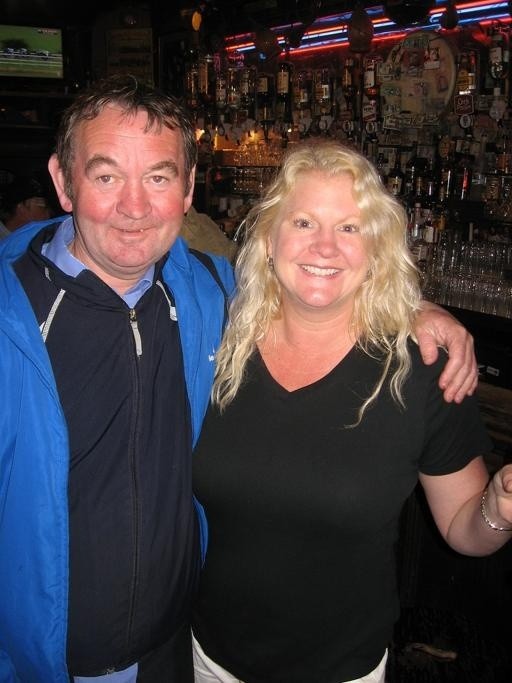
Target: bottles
[185,33,510,319]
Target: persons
[192,145,512,683]
[0,182,50,242]
[0,75,479,683]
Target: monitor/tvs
[0,20,66,82]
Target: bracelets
[480,480,510,534]
